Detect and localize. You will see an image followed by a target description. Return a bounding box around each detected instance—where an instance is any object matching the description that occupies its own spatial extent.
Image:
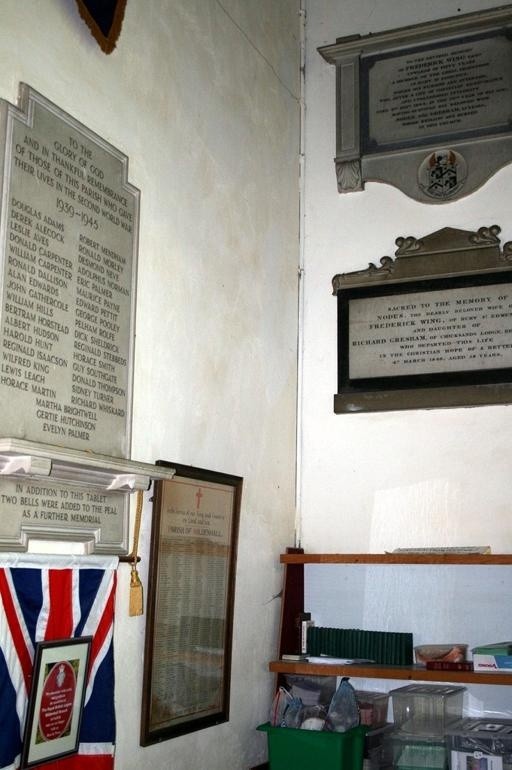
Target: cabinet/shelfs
[269,547,512,684]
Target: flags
[0,550,122,770]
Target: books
[298,611,312,654]
[425,659,473,672]
[301,620,316,654]
[282,652,311,661]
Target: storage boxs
[256,684,512,770]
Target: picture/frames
[21,635,92,769]
[139,460,243,747]
